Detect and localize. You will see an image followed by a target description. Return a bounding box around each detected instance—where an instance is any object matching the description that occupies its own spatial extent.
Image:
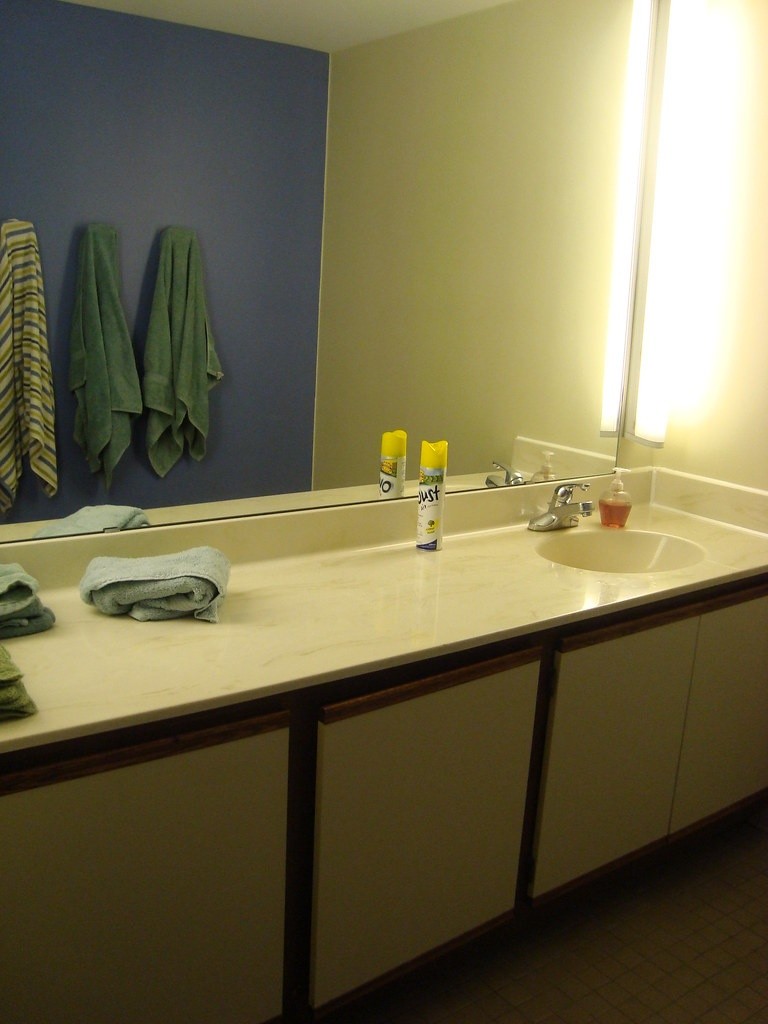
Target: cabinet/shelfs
[0,648,551,1024]
[536,595,767,899]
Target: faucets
[485,458,524,488]
[527,482,596,532]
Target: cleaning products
[377,429,409,501]
[415,439,449,553]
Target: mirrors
[0,0,674,554]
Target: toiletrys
[598,467,634,530]
[529,449,558,483]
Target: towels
[0,641,39,724]
[138,223,226,480]
[67,216,144,494]
[0,214,59,517]
[30,503,153,541]
[0,562,57,641]
[76,544,232,625]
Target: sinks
[402,480,491,498]
[533,528,710,576]
